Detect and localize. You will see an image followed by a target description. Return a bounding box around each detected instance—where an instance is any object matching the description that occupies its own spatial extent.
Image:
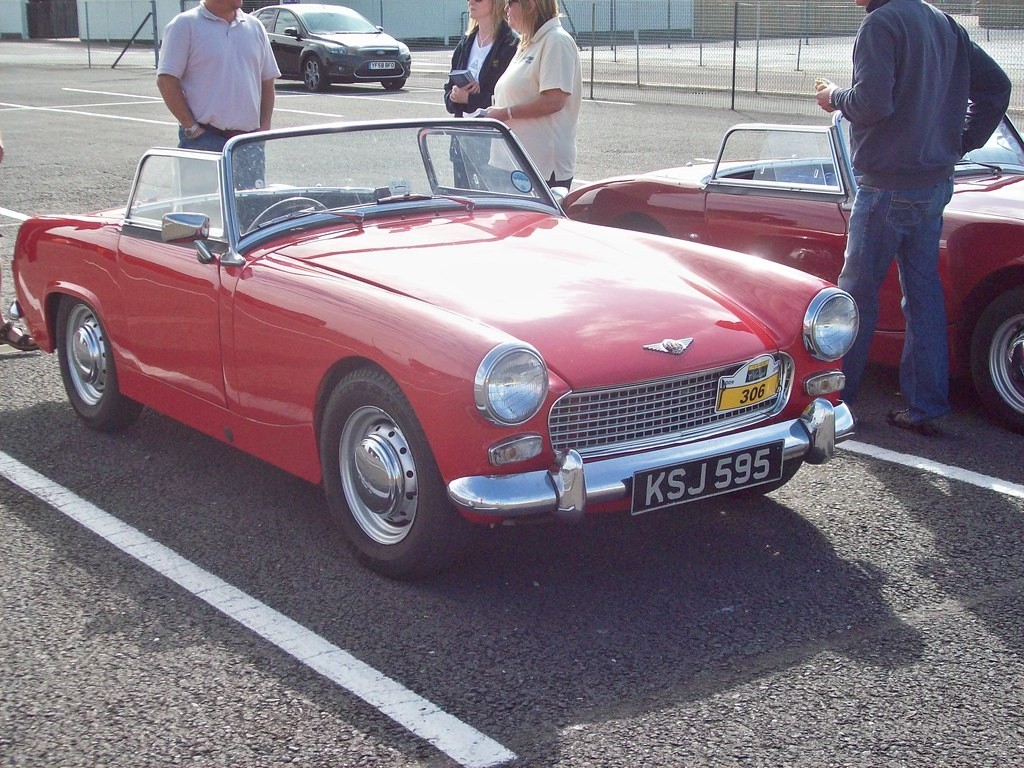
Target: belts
[198,122,260,140]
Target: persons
[488,0,582,196]
[444,1,521,188]
[812,1,1011,425]
[156,0,282,230]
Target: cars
[247,5,411,90]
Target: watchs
[184,123,199,136]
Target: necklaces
[479,33,490,46]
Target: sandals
[0,321,40,351]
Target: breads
[814,77,831,91]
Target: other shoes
[886,408,946,437]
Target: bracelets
[829,87,837,105]
[506,105,513,120]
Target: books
[448,70,480,95]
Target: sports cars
[8,120,858,572]
[559,104,1023,431]
